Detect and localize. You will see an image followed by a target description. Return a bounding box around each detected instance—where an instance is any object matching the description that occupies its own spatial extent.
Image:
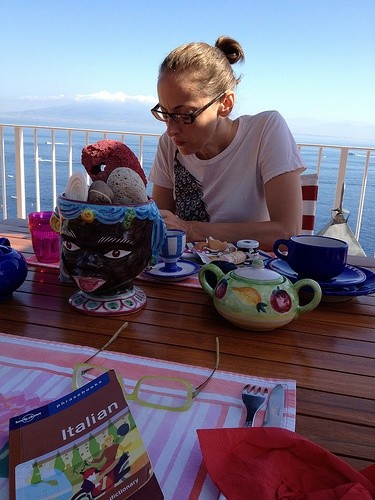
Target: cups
[272,234,349,282]
[26,211,61,263]
[57,192,154,317]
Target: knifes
[261,384,285,428]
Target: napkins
[196,427,375,500]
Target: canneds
[237,240,259,265]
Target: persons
[147,36,307,252]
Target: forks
[216,384,268,500]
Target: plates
[263,256,375,303]
[145,259,201,282]
[267,257,366,287]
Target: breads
[219,251,246,265]
[193,237,228,252]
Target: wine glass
[157,229,186,273]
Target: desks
[0,233,375,472]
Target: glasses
[73,321,220,412]
[150,92,226,125]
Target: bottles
[236,239,260,264]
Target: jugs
[198,259,322,332]
[0,238,29,293]
